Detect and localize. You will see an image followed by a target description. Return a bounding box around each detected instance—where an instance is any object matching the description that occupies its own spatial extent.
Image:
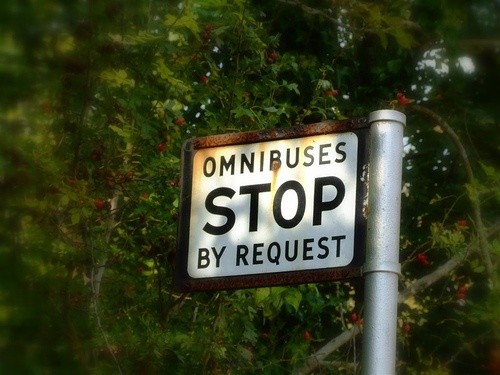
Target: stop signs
[173,117,367,291]
